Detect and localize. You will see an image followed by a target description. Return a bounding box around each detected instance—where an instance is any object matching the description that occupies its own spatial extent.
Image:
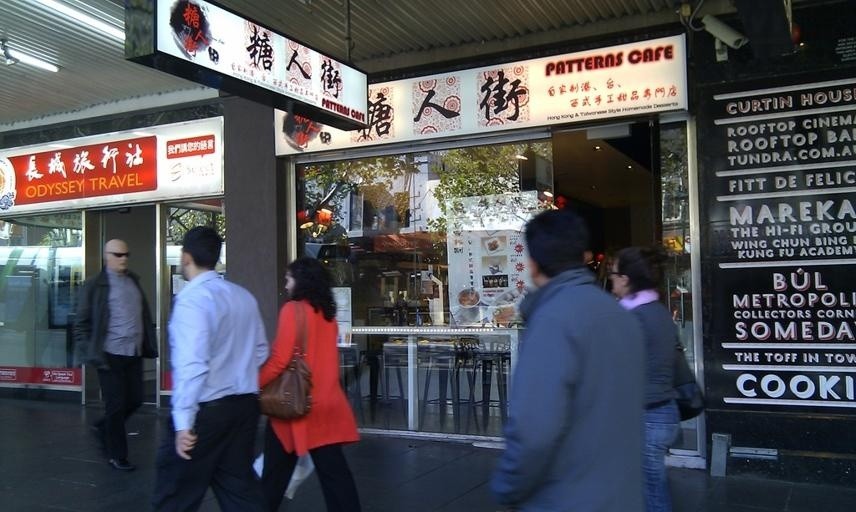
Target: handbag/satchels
[258,300,313,420]
[676,379,706,422]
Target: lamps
[36,0,126,40]
[7,47,59,73]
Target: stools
[419,348,479,434]
[352,350,407,427]
[465,349,511,433]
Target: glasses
[107,251,129,258]
[607,269,625,276]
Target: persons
[147,226,270,512]
[492,214,644,511]
[259,257,362,512]
[610,245,680,512]
[73,239,160,472]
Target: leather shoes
[108,458,136,472]
